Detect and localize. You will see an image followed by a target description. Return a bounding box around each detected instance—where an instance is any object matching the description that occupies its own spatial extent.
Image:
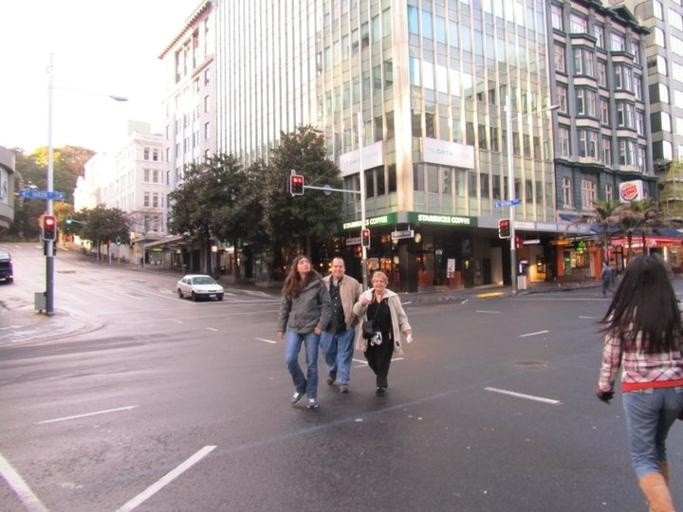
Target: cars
[175,274,223,301]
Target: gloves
[596,390,614,401]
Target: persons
[353,271,413,395]
[276,255,333,409]
[596,255,682,512]
[599,262,614,296]
[319,256,361,393]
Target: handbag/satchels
[363,320,373,339]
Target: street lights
[503,89,561,296]
[43,86,128,318]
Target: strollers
[0,252,13,283]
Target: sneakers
[376,386,387,395]
[292,392,303,404]
[339,385,348,393]
[307,398,319,408]
[327,372,336,385]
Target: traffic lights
[66,214,71,224]
[42,215,56,242]
[361,229,370,247]
[498,218,511,239]
[290,175,304,196]
